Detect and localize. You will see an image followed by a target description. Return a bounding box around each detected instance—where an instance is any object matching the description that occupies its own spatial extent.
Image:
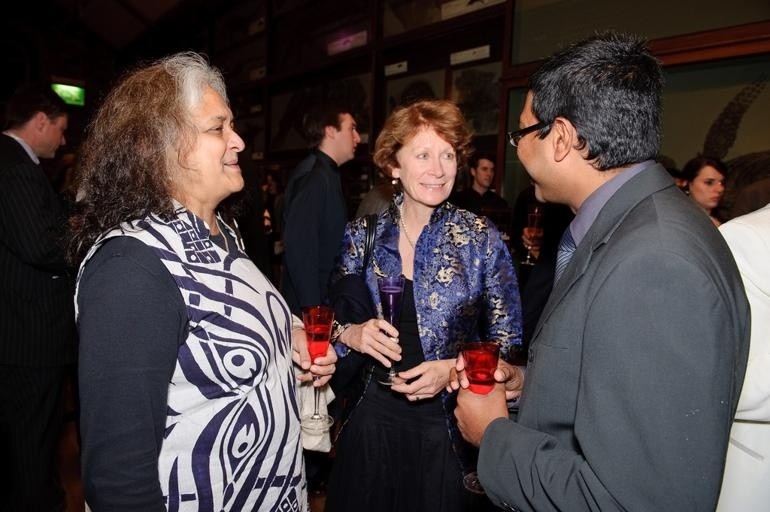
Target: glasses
[508,118,555,147]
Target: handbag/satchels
[361,214,379,281]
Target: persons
[455,149,509,236]
[281,108,363,308]
[443,30,752,511]
[673,146,727,233]
[1,84,71,233]
[71,50,338,512]
[320,98,523,509]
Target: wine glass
[301,307,334,429]
[377,278,407,385]
[462,342,500,492]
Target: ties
[553,229,576,286]
